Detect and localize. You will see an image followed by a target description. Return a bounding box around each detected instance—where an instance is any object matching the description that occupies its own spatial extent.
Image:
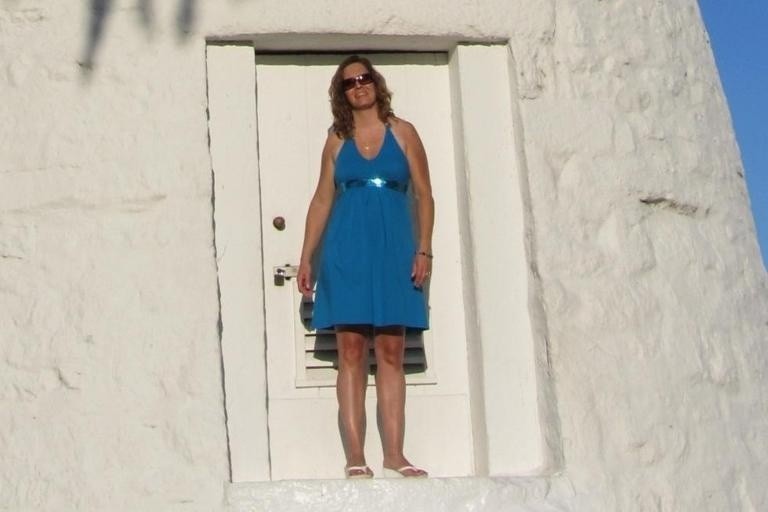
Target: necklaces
[356,124,384,150]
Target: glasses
[342,72,375,91]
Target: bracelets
[414,250,434,260]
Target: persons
[296,55,435,479]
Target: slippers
[345,464,373,478]
[383,464,427,479]
